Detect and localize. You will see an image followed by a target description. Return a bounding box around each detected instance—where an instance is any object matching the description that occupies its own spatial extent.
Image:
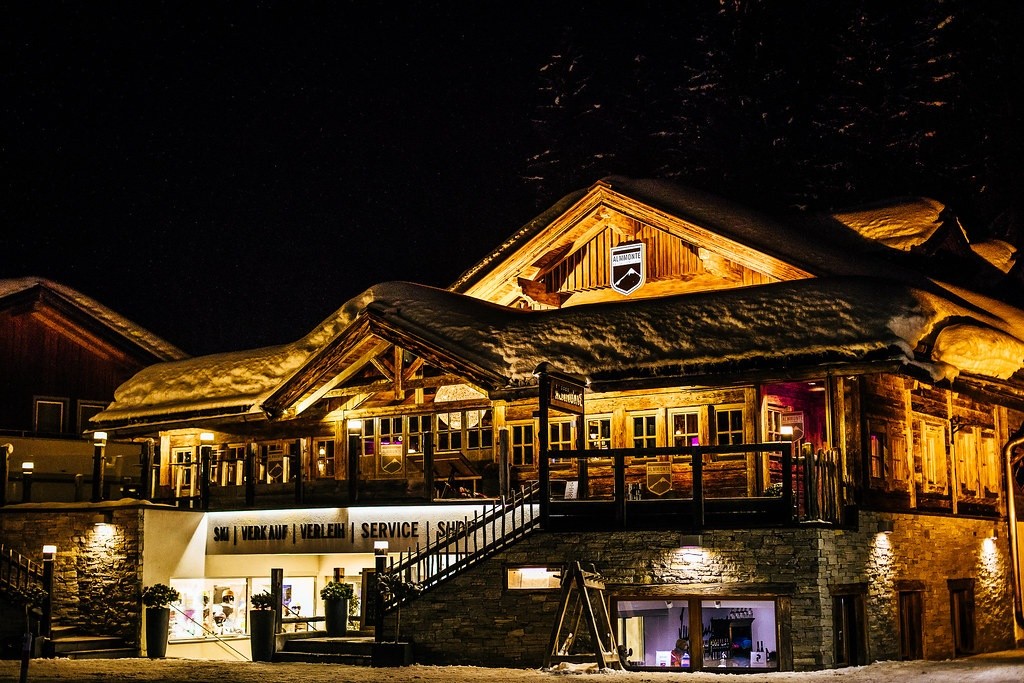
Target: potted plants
[140,582,179,658]
[320,581,354,636]
[251,593,276,661]
